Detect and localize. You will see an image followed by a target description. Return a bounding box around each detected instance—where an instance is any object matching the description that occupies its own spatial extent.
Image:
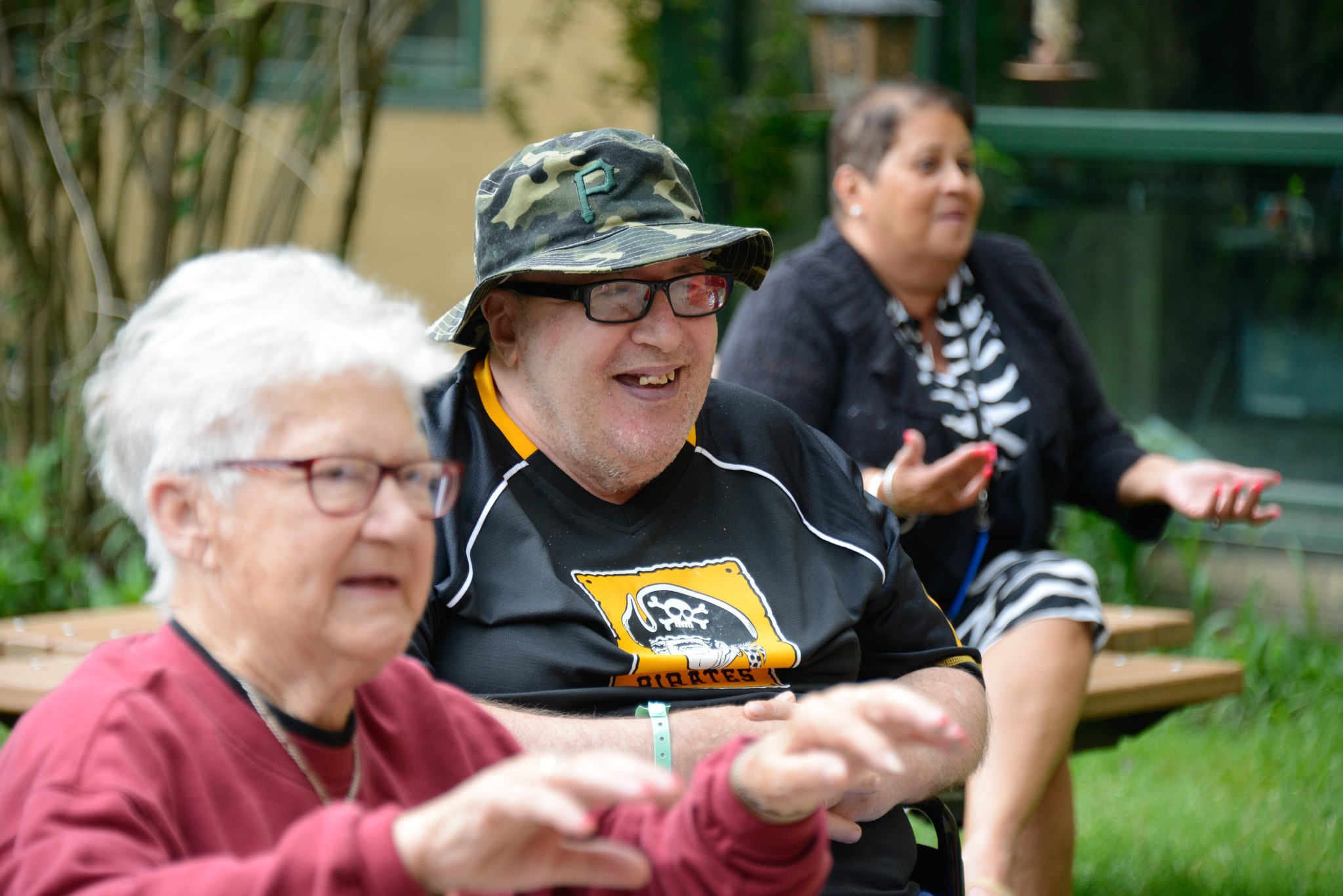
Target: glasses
[496,271,736,324]
[186,452,464,520]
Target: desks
[0,603,1247,752]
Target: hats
[425,127,774,344]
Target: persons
[719,75,1281,896]
[419,126,990,896]
[0,245,966,896]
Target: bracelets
[633,696,673,783]
[864,473,917,537]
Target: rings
[1210,516,1223,531]
